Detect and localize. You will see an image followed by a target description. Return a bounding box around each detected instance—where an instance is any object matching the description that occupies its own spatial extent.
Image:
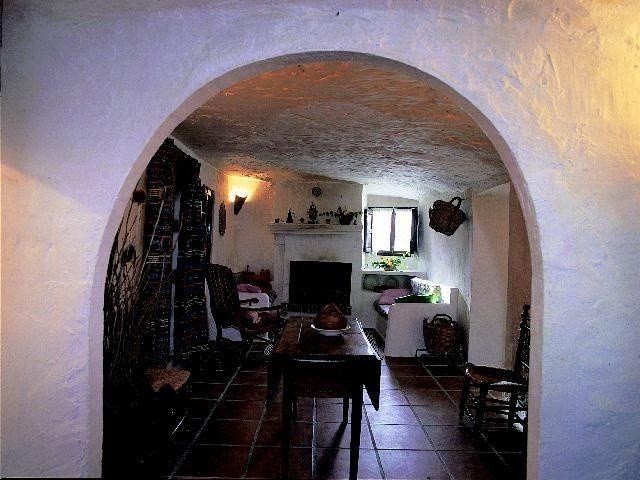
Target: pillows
[378,286,433,308]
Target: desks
[268,316,384,479]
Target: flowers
[371,250,411,271]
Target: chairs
[459,303,531,442]
[205,259,283,360]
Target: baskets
[422,313,464,355]
[430,196,467,236]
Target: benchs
[373,277,458,357]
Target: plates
[310,322,351,337]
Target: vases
[385,266,395,270]
